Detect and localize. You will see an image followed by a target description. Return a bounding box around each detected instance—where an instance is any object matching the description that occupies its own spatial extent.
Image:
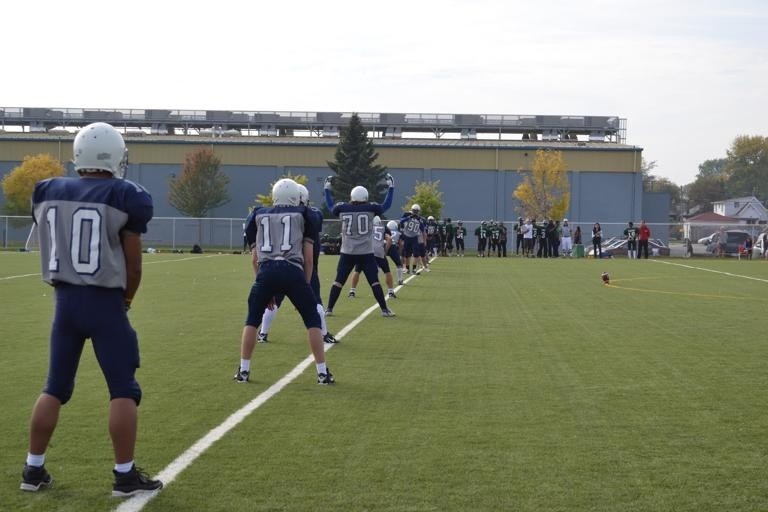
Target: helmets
[348,185,370,203]
[373,202,437,231]
[479,218,505,228]
[517,216,569,224]
[68,120,129,175]
[270,177,301,207]
[296,184,310,207]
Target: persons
[347,215,396,299]
[256,184,339,343]
[233,178,335,386]
[320,232,342,255]
[19,121,162,497]
[624,222,639,260]
[743,238,752,260]
[399,203,582,275]
[637,219,650,259]
[591,223,602,258]
[383,221,405,285]
[323,173,396,317]
[717,226,728,258]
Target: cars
[584,235,673,258]
[697,228,768,257]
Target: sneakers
[256,329,269,344]
[321,331,339,344]
[232,366,250,383]
[325,308,332,316]
[516,252,574,259]
[111,461,164,497]
[430,249,466,257]
[17,461,54,491]
[346,258,431,301]
[381,308,396,317]
[314,365,336,386]
[474,250,508,258]
